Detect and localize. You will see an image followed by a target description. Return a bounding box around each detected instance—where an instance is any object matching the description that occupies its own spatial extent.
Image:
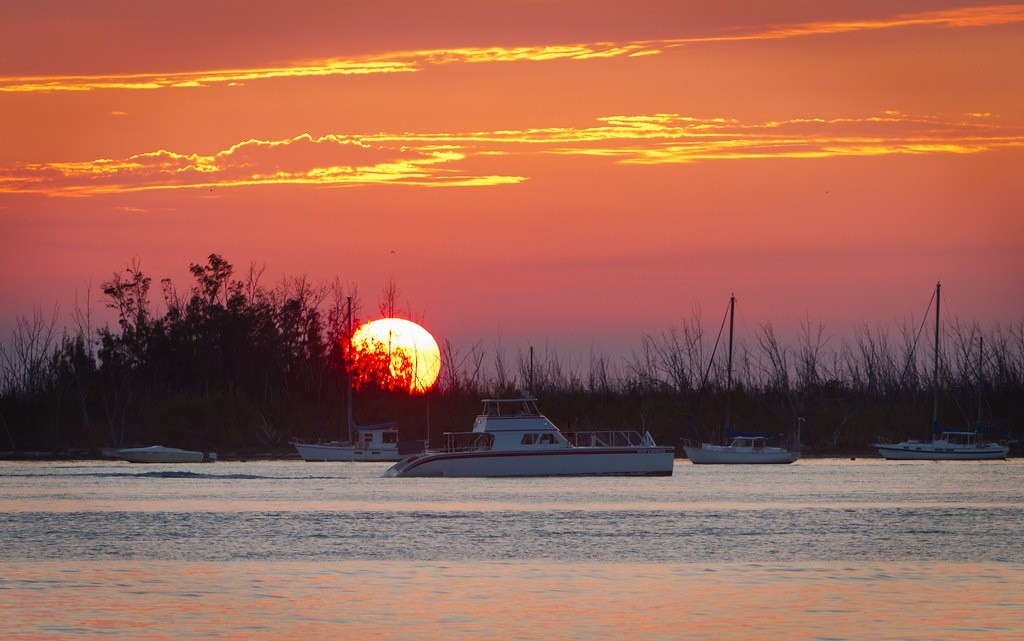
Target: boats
[116,445,204,463]
[384,347,675,479]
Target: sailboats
[287,296,436,462]
[870,280,1010,460]
[679,293,803,465]
